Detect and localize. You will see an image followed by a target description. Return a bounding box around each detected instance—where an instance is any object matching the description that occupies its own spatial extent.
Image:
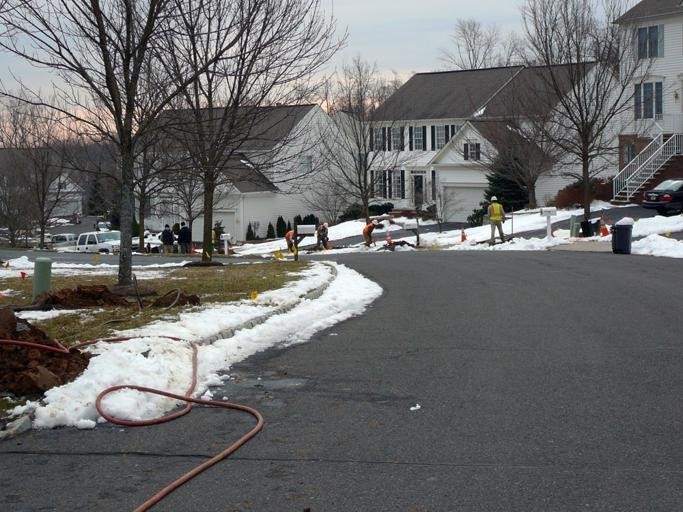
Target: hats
[165,224,169,228]
[181,222,185,226]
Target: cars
[0,221,179,253]
[642,177,683,214]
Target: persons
[144,229,152,238]
[212,229,217,254]
[487,195,506,245]
[179,222,191,253]
[317,222,330,250]
[285,230,295,252]
[363,219,378,247]
[161,224,174,253]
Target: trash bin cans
[34,257,51,295]
[612,225,632,254]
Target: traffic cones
[386,232,392,246]
[191,242,195,255]
[461,226,466,242]
[600,213,609,236]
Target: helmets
[491,196,497,200]
[323,223,328,228]
[372,220,378,225]
